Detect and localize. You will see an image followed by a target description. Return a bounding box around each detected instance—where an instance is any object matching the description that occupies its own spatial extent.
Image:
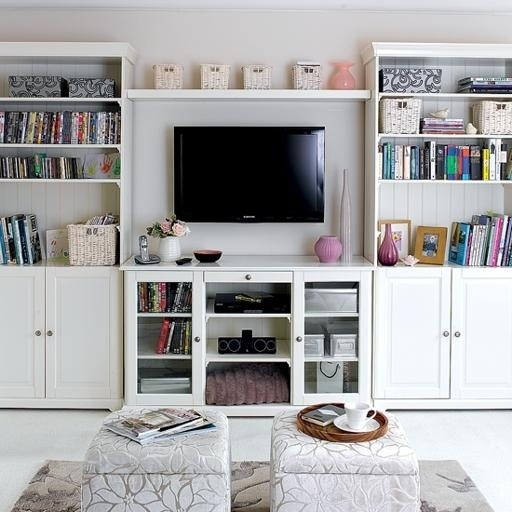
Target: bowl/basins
[194,249,222,263]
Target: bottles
[141,237,150,261]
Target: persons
[425,235,437,256]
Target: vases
[328,60,358,90]
[156,235,181,262]
[314,235,342,263]
[340,169,352,263]
[377,223,398,266]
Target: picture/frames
[413,226,448,265]
[378,219,413,263]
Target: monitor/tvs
[174,125,325,223]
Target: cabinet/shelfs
[360,41,512,413]
[0,41,138,413]
[119,253,374,417]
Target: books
[138,282,191,312]
[1,155,77,179]
[140,368,191,393]
[0,111,120,143]
[156,320,192,354]
[0,214,42,265]
[301,405,345,427]
[379,139,512,180]
[422,117,465,135]
[458,76,512,95]
[449,213,512,266]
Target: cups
[344,399,377,430]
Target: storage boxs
[304,333,325,357]
[304,288,357,314]
[68,78,117,98]
[379,67,442,92]
[7,75,63,98]
[325,332,358,358]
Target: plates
[333,415,381,433]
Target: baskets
[153,64,184,90]
[200,64,230,89]
[471,100,511,135]
[67,223,120,266]
[379,98,422,134]
[241,65,271,90]
[291,65,322,89]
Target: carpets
[9,460,494,512]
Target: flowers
[144,213,191,238]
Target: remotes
[176,257,192,265]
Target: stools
[269,408,424,512]
[81,404,231,511]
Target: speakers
[217,337,277,354]
